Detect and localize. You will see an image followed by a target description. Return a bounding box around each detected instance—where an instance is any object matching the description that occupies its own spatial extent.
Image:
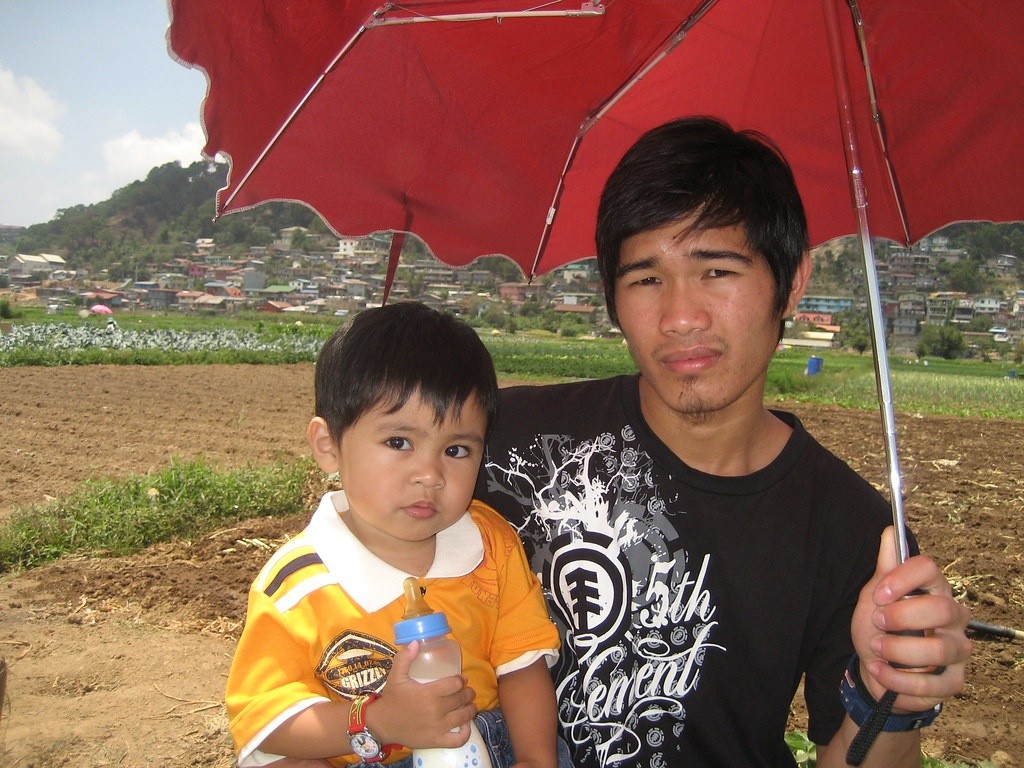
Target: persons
[224,297,562,768]
[260,116,975,768]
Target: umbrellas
[164,1,1024,674]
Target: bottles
[393,577,493,768]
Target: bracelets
[836,653,944,733]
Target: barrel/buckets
[808,357,823,376]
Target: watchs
[344,691,404,764]
